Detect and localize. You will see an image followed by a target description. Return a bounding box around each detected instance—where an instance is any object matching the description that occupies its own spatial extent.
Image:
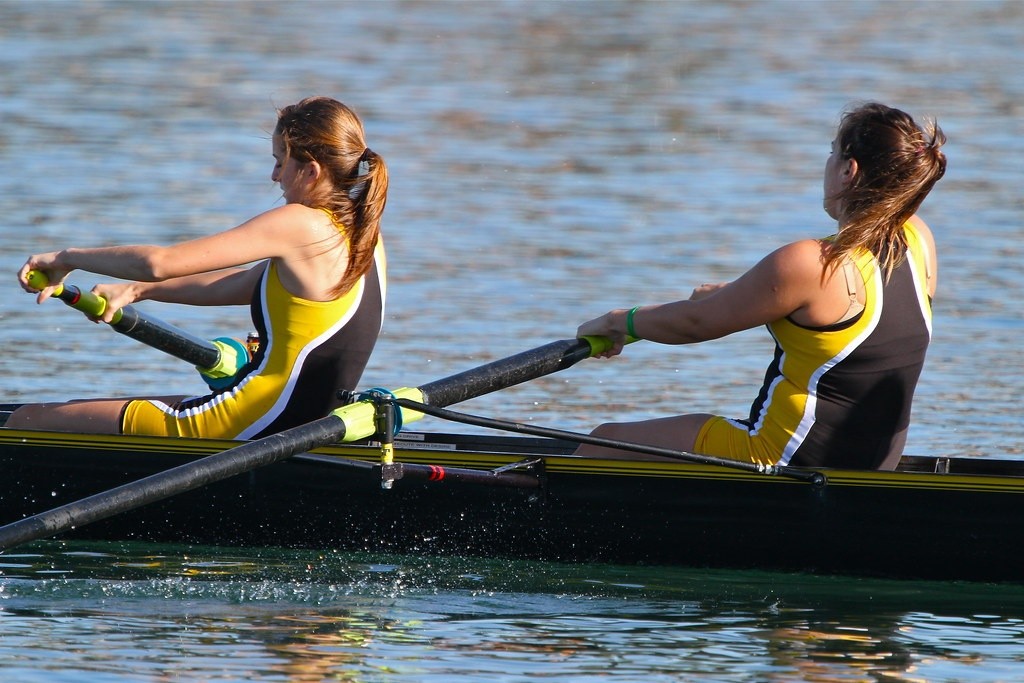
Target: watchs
[627,306,641,339]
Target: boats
[0,402,1024,583]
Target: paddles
[25,268,260,381]
[0,335,641,555]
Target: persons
[571,101,948,475]
[4,98,388,440]
